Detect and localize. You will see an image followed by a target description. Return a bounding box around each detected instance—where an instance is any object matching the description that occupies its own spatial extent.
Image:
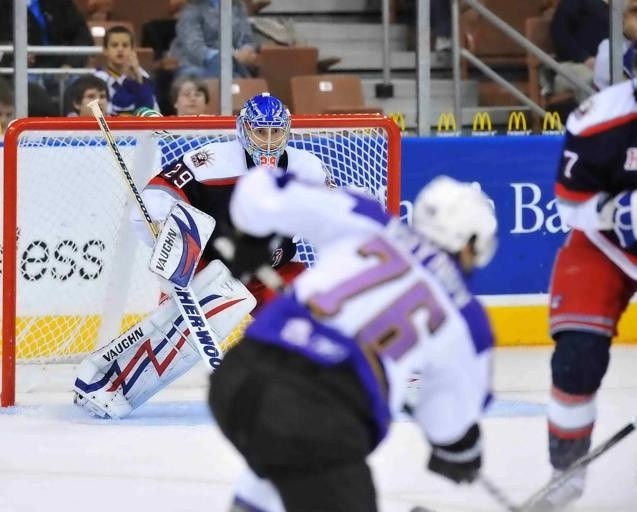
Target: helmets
[236,91,292,168]
[409,172,499,269]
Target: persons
[0,1,262,147]
[72,93,330,418]
[394,1,636,130]
[209,163,500,512]
[522,44,636,511]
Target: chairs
[451,0,584,133]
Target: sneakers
[536,461,584,512]
